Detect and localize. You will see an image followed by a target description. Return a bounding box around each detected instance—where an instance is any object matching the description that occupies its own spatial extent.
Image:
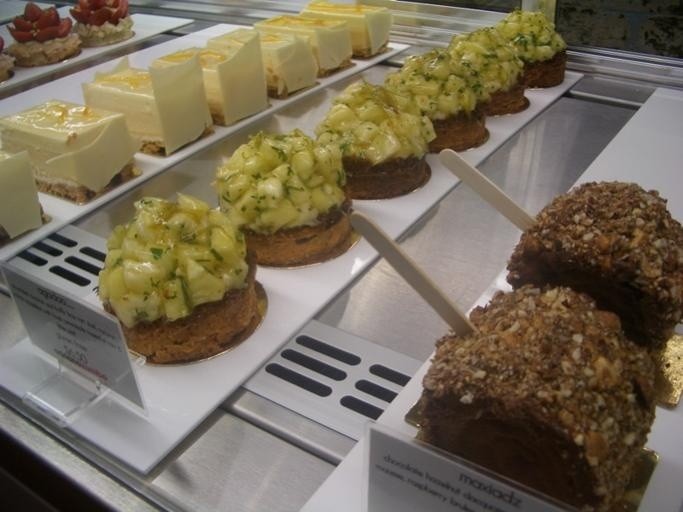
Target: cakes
[208,127,353,268]
[253,14,356,80]
[97,191,266,364]
[148,31,269,127]
[313,78,436,200]
[2,2,82,66]
[0,35,16,83]
[298,0,393,61]
[505,179,682,412]
[403,284,657,512]
[0,98,141,205]
[68,0,135,48]
[206,27,318,100]
[0,149,45,246]
[80,51,214,157]
[444,26,527,116]
[492,6,567,88]
[381,49,489,153]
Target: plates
[0,46,588,480]
[3,22,414,272]
[2,5,196,102]
[296,85,679,512]
